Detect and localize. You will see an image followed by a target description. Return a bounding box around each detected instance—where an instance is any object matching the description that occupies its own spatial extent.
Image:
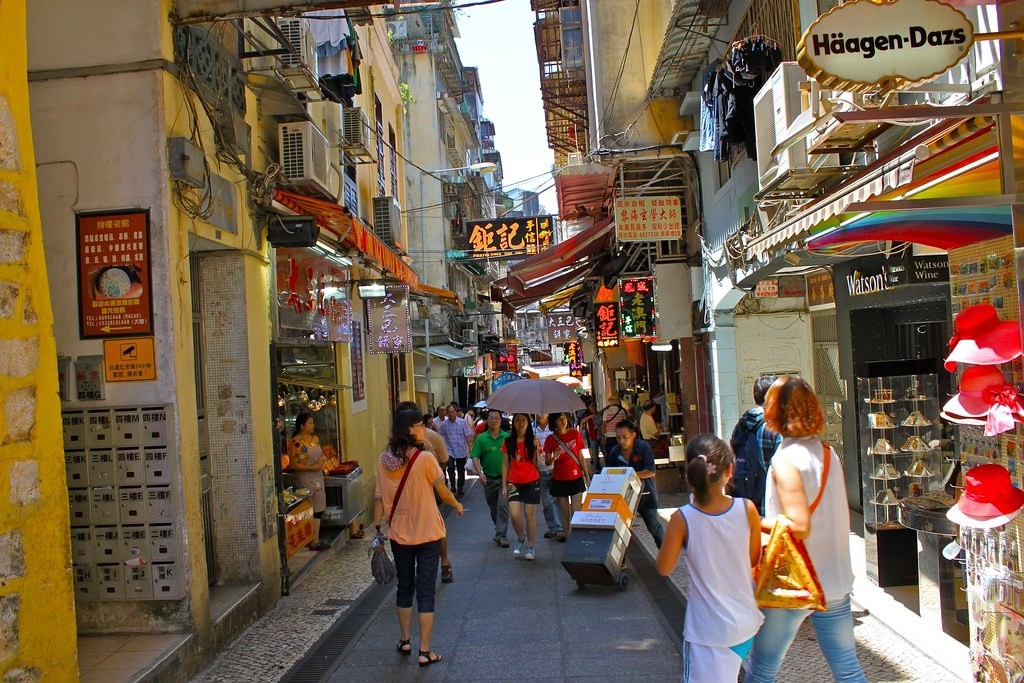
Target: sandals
[396,640,412,656]
[418,649,443,667]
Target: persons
[745,375,870,683]
[580,392,660,474]
[608,420,666,550]
[374,401,464,667]
[286,411,333,551]
[655,434,762,683]
[731,375,783,516]
[500,413,546,560]
[532,412,591,543]
[422,401,487,496]
[469,409,511,548]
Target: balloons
[497,354,515,363]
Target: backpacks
[728,417,771,504]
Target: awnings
[643,0,733,101]
[490,218,615,321]
[276,372,353,391]
[745,102,1024,268]
[554,163,618,221]
[272,190,419,291]
[411,285,462,312]
[412,344,476,377]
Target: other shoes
[497,536,510,548]
[544,529,556,539]
[441,564,454,583]
[450,487,457,494]
[525,544,536,560]
[590,456,594,464]
[457,491,464,500]
[512,535,528,558]
[555,530,567,542]
[309,541,332,550]
[493,534,500,541]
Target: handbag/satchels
[463,458,482,475]
[750,514,827,613]
[368,532,395,585]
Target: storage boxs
[668,446,685,462]
[561,468,642,587]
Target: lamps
[357,284,387,299]
[270,216,320,248]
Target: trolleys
[572,480,645,591]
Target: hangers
[733,23,784,49]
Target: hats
[929,294,1023,531]
[608,395,618,404]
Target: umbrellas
[473,400,487,409]
[487,379,587,436]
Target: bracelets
[585,471,589,475]
[552,456,557,461]
[501,484,507,489]
[476,471,484,476]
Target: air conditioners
[280,17,318,88]
[368,196,400,248]
[463,329,474,341]
[279,122,334,198]
[345,107,371,156]
